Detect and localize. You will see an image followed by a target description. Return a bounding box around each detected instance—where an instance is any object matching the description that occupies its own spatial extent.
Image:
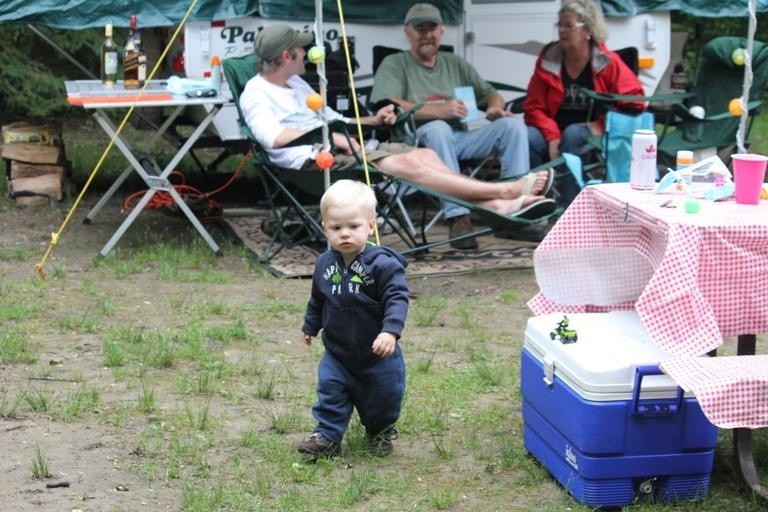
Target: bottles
[211,56,224,93]
[121,13,146,90]
[675,149,695,183]
[98,21,120,86]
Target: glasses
[555,20,587,31]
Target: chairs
[658,35,767,182]
[219,31,641,259]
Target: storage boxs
[521,307,719,502]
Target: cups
[731,154,768,206]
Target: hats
[254,23,314,63]
[404,2,444,27]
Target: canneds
[630,129,658,190]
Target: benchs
[669,355,767,499]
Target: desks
[533,180,768,498]
[61,78,229,263]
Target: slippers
[519,167,556,196]
[506,194,557,221]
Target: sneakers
[297,432,342,457]
[448,215,479,250]
[492,221,548,242]
[364,426,396,457]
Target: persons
[238,21,556,222]
[522,2,646,225]
[369,1,546,249]
[298,176,411,459]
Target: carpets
[220,206,541,277]
